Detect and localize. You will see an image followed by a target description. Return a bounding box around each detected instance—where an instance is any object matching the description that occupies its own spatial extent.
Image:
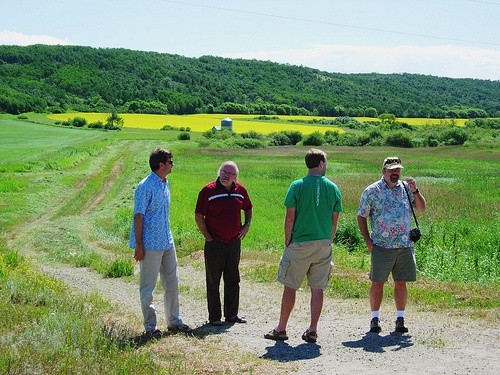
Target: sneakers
[396,318,408,332]
[371,318,381,331]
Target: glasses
[384,158,401,166]
[166,161,173,164]
[388,168,400,173]
[221,170,236,176]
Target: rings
[412,180,413,182]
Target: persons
[356,156,426,333]
[264,147,343,342]
[194,160,253,326]
[128,146,193,339]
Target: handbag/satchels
[409,228,420,242]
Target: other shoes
[225,317,247,323]
[210,319,223,326]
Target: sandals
[146,330,162,338]
[264,329,288,340]
[302,329,317,342]
[168,324,192,334]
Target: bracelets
[245,221,250,224]
[412,189,420,194]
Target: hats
[383,157,404,169]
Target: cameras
[410,228,421,242]
